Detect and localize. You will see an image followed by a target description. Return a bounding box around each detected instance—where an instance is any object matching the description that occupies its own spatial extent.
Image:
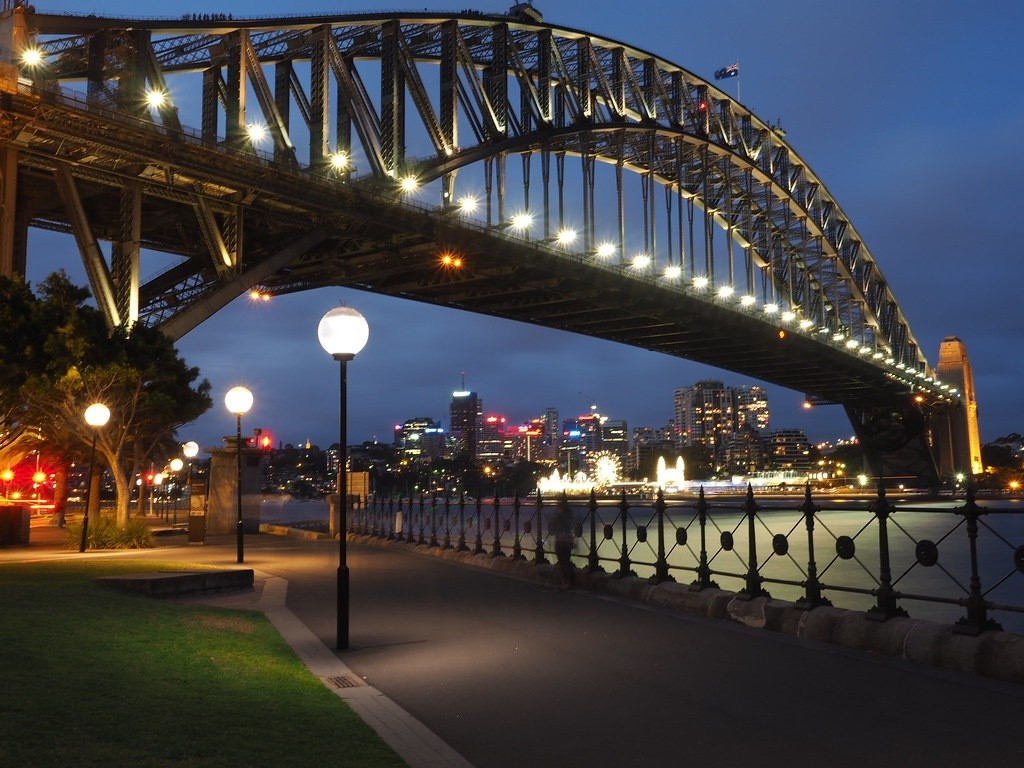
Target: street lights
[77,402,111,553]
[154,473,164,516]
[317,306,372,654]
[170,458,184,526]
[225,385,254,564]
[182,441,200,525]
